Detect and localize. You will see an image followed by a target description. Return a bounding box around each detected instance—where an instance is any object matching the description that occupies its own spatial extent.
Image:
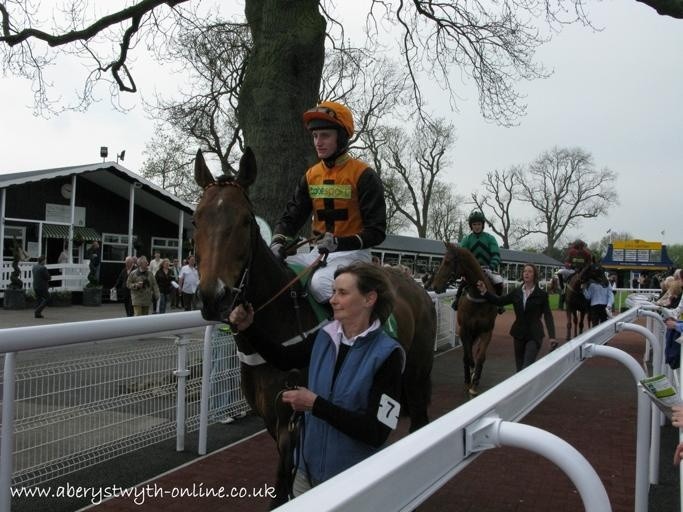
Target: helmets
[305,119,349,153]
[468,211,485,226]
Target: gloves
[316,233,363,254]
[269,233,291,263]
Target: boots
[452,280,467,311]
[493,282,504,314]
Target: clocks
[61,183,73,200]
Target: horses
[563,255,610,342]
[431,239,499,401]
[191,144,439,512]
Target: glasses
[306,107,354,139]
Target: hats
[302,102,353,137]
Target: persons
[476,262,559,372]
[114,250,200,316]
[632,267,683,467]
[226,258,407,503]
[84,241,100,260]
[17,244,31,263]
[32,256,51,318]
[553,240,618,345]
[269,100,387,318]
[57,245,68,285]
[451,211,507,315]
